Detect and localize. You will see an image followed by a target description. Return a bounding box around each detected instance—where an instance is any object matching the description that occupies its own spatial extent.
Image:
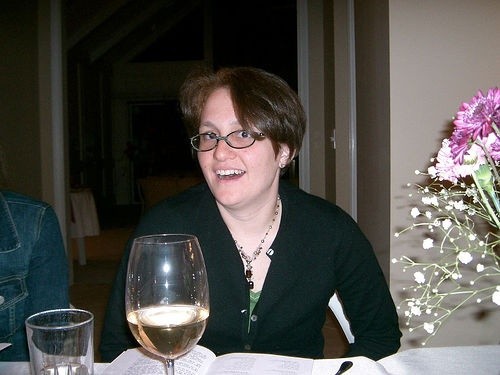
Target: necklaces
[220,196,281,291]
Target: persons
[103,67,401,363]
[0,195,71,362]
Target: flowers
[391,87,500,346]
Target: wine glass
[125,234,210,375]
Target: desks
[26,187,100,265]
[137,174,204,211]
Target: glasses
[190,129,265,152]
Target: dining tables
[1,362,113,375]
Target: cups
[25,309,94,375]
